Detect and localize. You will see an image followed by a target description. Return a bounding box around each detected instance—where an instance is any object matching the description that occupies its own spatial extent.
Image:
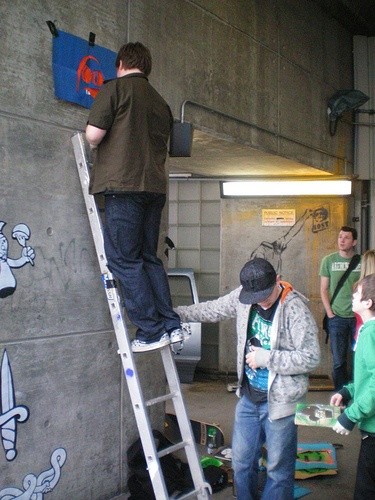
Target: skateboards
[164,413,224,449]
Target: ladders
[71,132,213,500]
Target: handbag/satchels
[127,430,186,500]
[321,312,328,333]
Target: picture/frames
[219,180,355,196]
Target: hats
[239,257,277,304]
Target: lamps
[327,89,371,136]
[169,121,194,157]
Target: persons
[319,226,362,393]
[174,258,321,500]
[85,41,183,352]
[351,249,375,351]
[330,273,375,500]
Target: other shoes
[116,328,184,354]
